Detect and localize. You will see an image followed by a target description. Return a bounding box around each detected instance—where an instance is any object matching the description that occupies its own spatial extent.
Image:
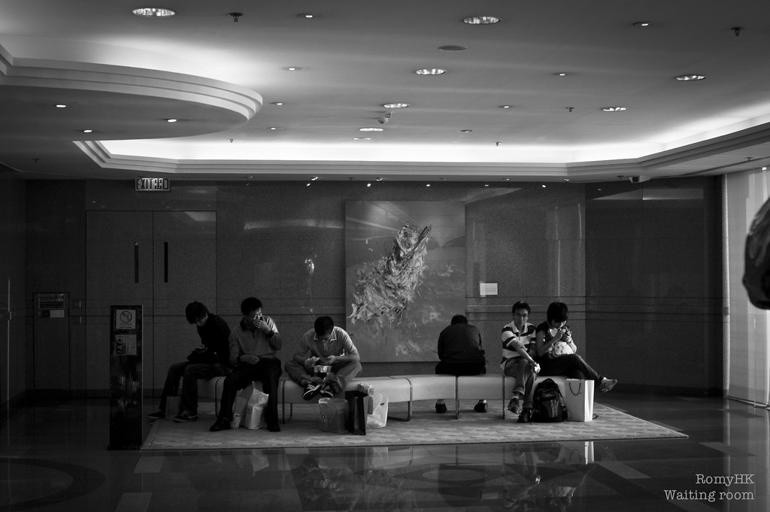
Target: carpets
[137,399,689,451]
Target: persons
[499,300,537,423]
[147,299,233,423]
[283,315,363,402]
[434,313,488,414]
[115,338,126,355]
[208,296,282,432]
[535,301,618,419]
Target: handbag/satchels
[165,394,182,420]
[314,396,349,435]
[473,332,488,375]
[344,387,372,436]
[242,381,269,430]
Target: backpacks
[545,328,578,360]
[533,378,570,423]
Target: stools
[181,374,569,425]
[156,441,564,472]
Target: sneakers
[599,376,618,394]
[208,418,282,434]
[317,383,335,398]
[473,401,489,412]
[517,406,532,423]
[301,383,322,401]
[434,401,447,414]
[146,409,199,423]
[507,397,520,414]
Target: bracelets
[265,329,274,339]
[567,338,574,345]
[236,354,242,364]
